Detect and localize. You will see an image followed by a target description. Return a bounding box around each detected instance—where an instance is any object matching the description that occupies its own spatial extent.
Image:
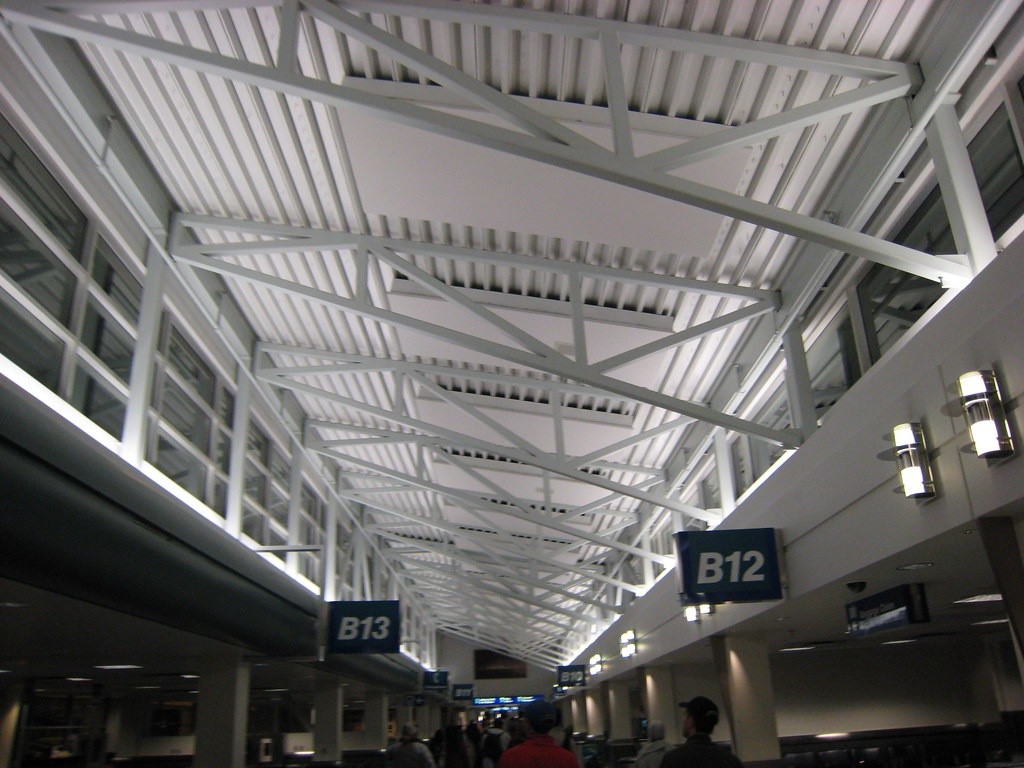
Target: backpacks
[481,731,504,762]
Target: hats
[647,719,664,741]
[402,723,417,736]
[525,701,556,727]
[679,696,719,726]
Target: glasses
[679,710,693,721]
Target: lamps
[893,419,936,506]
[958,362,1016,471]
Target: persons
[634,696,747,768]
[386,704,585,768]
